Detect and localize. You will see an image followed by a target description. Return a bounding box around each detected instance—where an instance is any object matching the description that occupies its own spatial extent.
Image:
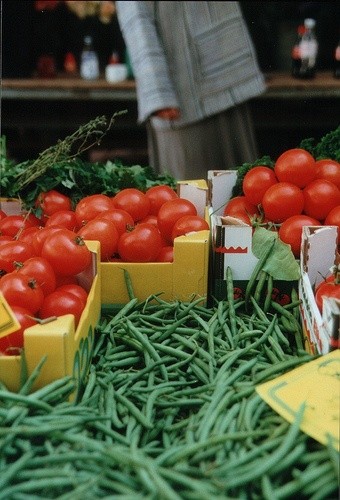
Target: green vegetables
[0,109,181,219]
[230,124,340,198]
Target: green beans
[0,235,340,500]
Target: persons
[115,0,267,183]
[1,0,340,166]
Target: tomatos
[222,146,340,319]
[0,187,209,354]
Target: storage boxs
[0,172,340,401]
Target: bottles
[299,18,318,79]
[291,27,307,77]
[79,36,100,81]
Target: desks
[3,73,339,165]
